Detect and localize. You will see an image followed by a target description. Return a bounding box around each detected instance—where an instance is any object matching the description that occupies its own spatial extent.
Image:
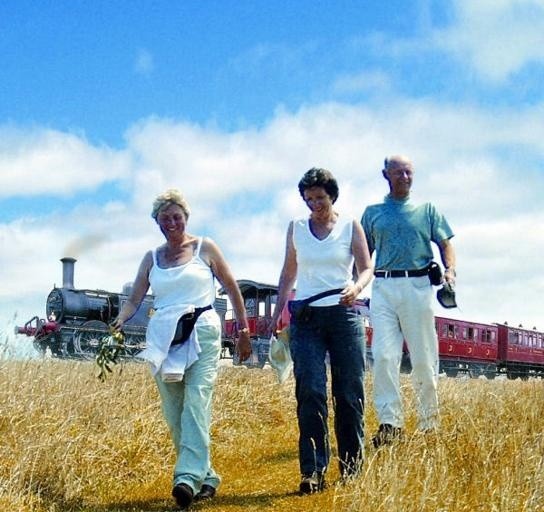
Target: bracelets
[236,328,248,333]
[445,267,456,276]
[355,282,363,293]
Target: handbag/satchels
[288,299,311,324]
[170,304,213,346]
[429,261,444,286]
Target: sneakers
[298,475,325,494]
[195,484,215,501]
[373,425,401,448]
[174,484,193,509]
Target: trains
[11,255,543,382]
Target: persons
[108,188,251,506]
[351,153,458,452]
[264,167,375,495]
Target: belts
[374,265,428,278]
